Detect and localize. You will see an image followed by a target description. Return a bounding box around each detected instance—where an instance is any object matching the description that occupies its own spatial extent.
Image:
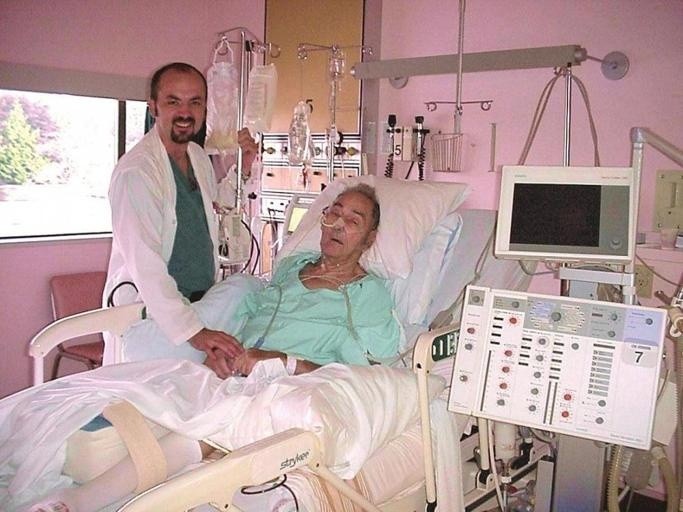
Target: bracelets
[284,354,297,377]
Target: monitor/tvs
[495,165,633,263]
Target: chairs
[49,271,107,379]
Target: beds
[0,207,559,512]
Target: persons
[28,179,404,512]
[94,61,262,361]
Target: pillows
[232,363,446,470]
[120,274,264,364]
[387,212,463,326]
[272,176,468,279]
